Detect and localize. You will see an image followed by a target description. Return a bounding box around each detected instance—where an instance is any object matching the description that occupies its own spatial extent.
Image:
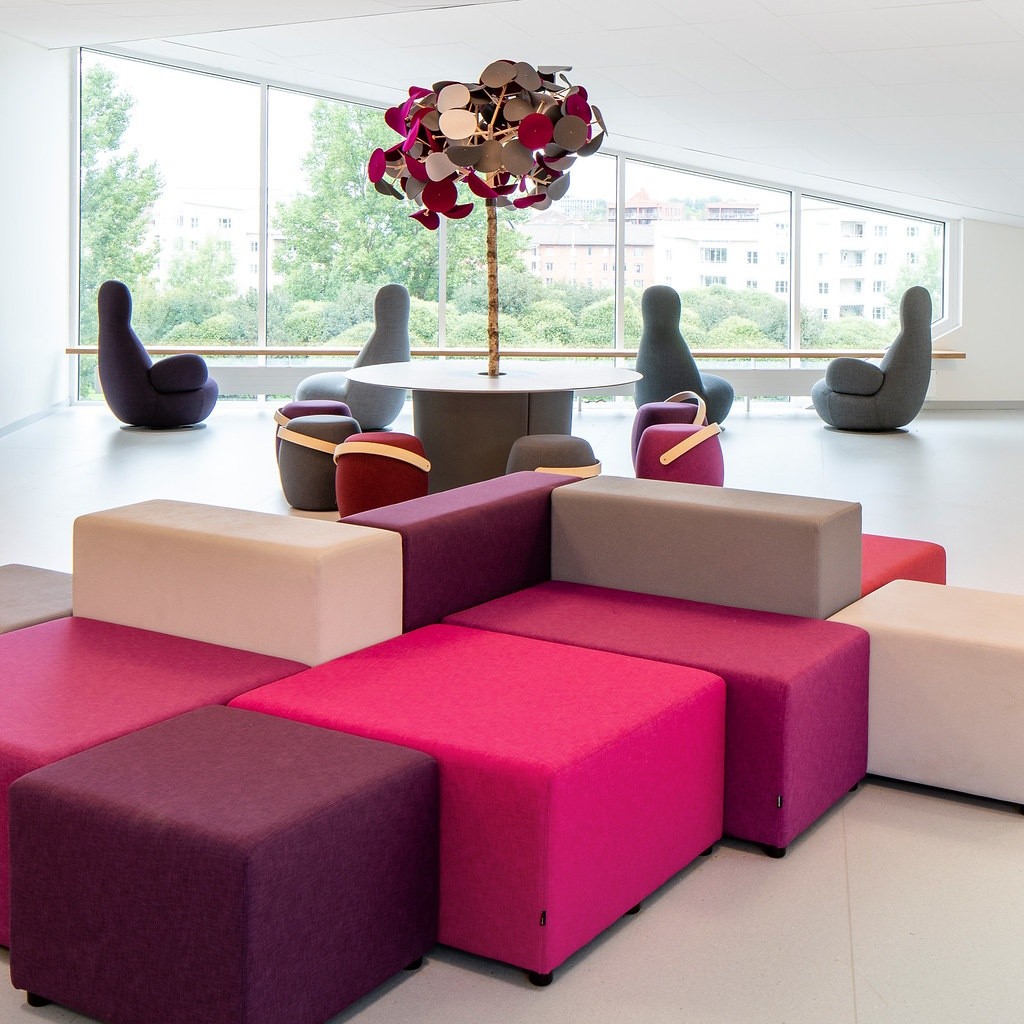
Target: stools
[0,401,1024,1024]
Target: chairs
[97,280,219,428]
[812,287,931,433]
[634,285,734,427]
[294,283,411,424]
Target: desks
[343,358,642,479]
